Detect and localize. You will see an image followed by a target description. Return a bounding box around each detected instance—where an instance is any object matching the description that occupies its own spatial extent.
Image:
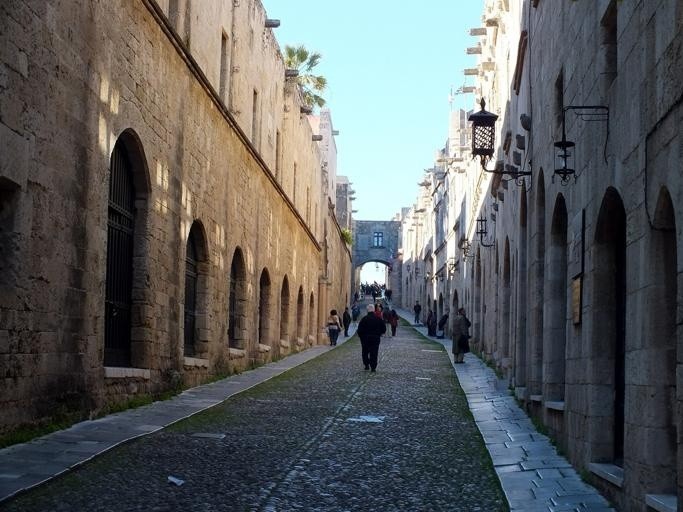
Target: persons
[326,310,342,346]
[426,310,437,336]
[343,307,351,337]
[388,309,399,337]
[452,308,471,363]
[358,304,386,372]
[351,280,392,320]
[414,301,421,323]
[436,312,449,339]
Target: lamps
[550,105,610,187]
[475,214,495,248]
[468,96,534,185]
